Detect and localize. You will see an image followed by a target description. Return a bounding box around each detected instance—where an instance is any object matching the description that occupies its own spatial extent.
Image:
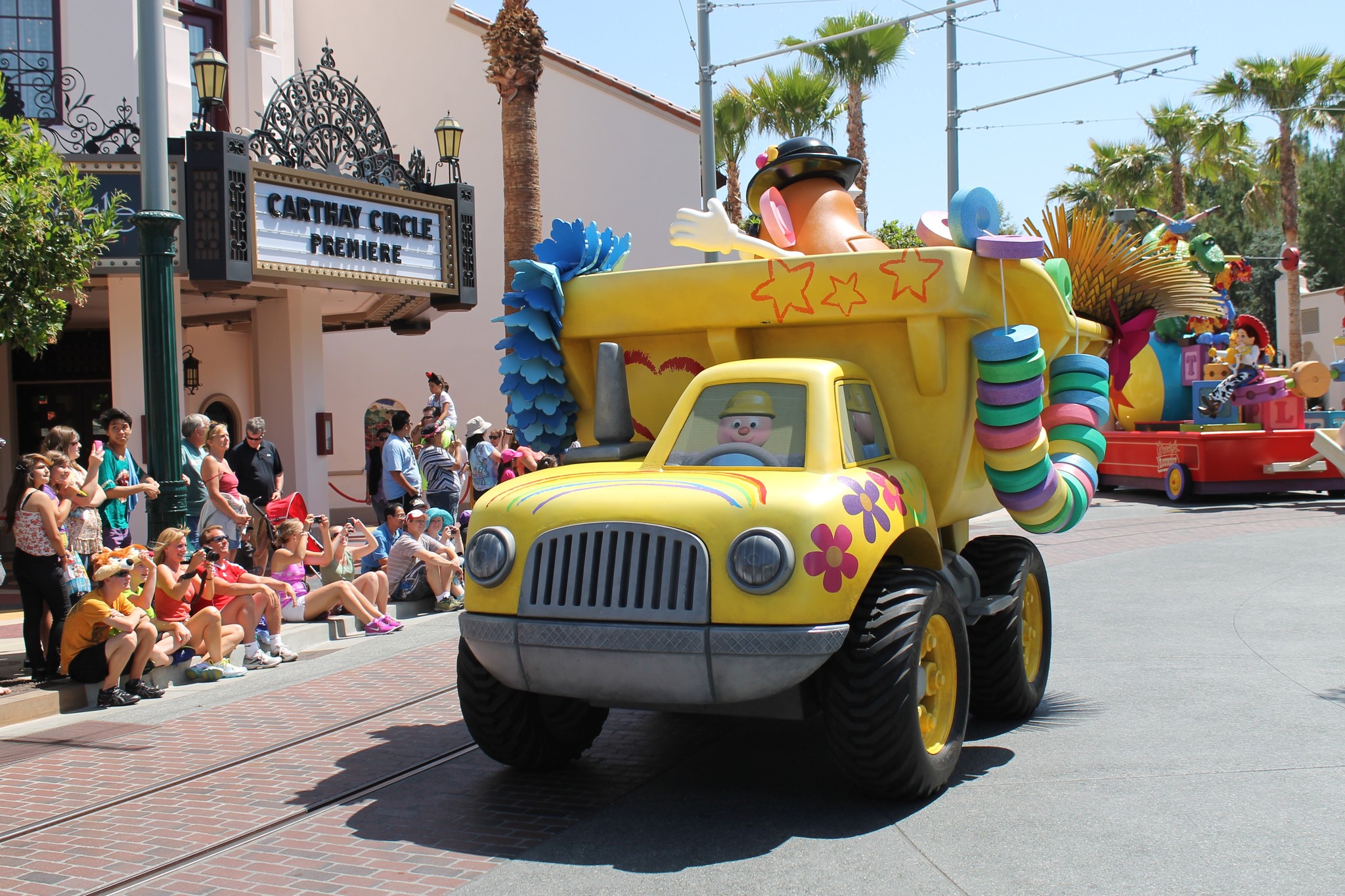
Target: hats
[459,510,472,525]
[500,449,523,463]
[424,507,454,534]
[421,423,444,438]
[405,509,429,523]
[404,498,429,513]
[465,415,492,439]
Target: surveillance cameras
[1109,208,1135,222]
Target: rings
[202,557,204,560]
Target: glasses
[379,436,388,441]
[207,422,226,440]
[423,415,436,420]
[331,533,349,540]
[394,515,406,519]
[246,431,264,440]
[488,435,500,440]
[113,570,129,577]
[207,535,229,542]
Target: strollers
[252,492,321,582]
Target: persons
[181,413,210,550]
[365,372,581,525]
[229,416,284,576]
[114,544,196,668]
[320,517,401,630]
[361,498,473,612]
[3,407,161,687]
[1197,314,1269,419]
[670,136,890,259]
[235,526,269,575]
[61,546,164,707]
[148,528,248,682]
[1136,205,1222,260]
[272,513,404,636]
[191,525,298,670]
[197,421,252,563]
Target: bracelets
[59,554,66,558]
[206,576,214,580]
[300,533,309,537]
[275,489,283,496]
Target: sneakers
[125,679,165,699]
[170,645,196,669]
[365,616,403,636]
[270,643,298,663]
[434,596,465,612]
[242,649,282,670]
[185,659,224,682]
[96,685,141,708]
[215,657,248,678]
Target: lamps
[428,109,468,187]
[182,344,203,396]
[36,303,72,342]
[190,38,243,136]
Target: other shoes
[31,667,67,683]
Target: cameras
[503,429,514,434]
[199,545,220,562]
[446,527,456,534]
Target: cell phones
[313,515,322,524]
[451,435,454,441]
[94,440,103,455]
[346,518,354,532]
